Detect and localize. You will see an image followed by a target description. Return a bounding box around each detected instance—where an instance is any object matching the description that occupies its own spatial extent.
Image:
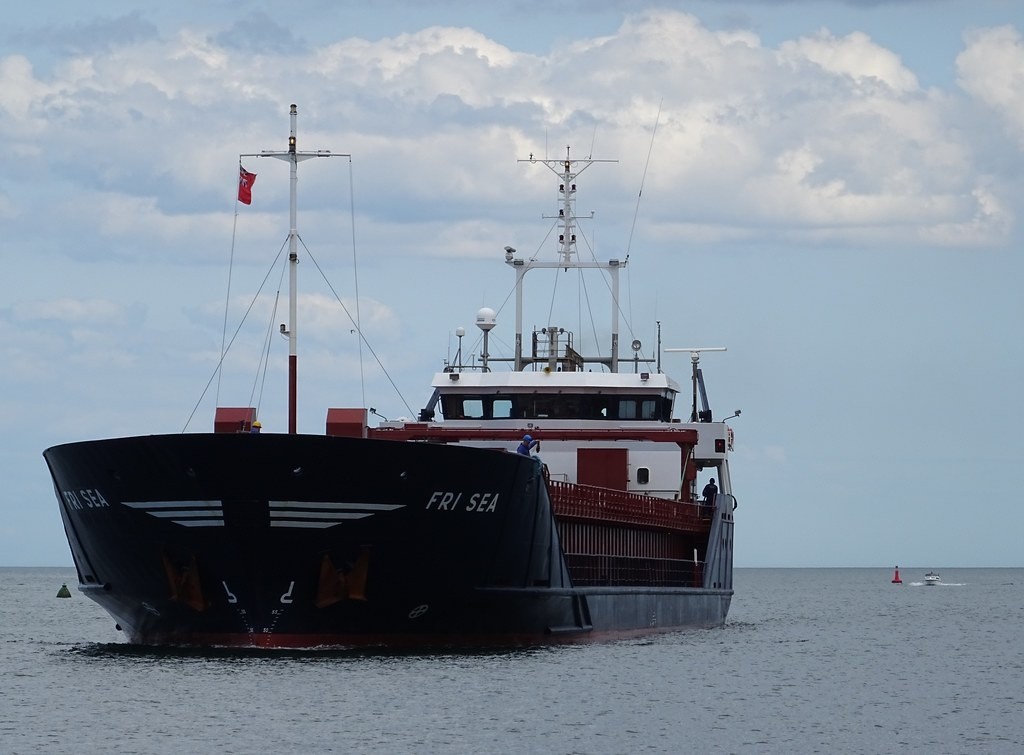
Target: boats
[923,570,942,587]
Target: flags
[238,161,259,208]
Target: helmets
[523,435,532,441]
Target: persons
[517,433,539,456]
[701,475,719,505]
[249,420,260,433]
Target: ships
[42,102,744,658]
